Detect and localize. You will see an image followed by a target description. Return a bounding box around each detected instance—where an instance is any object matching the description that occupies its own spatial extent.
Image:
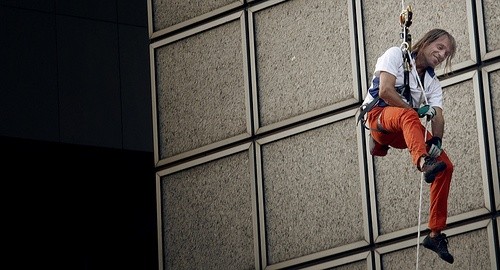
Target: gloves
[416,105,437,118]
[427,137,442,158]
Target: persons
[363,29,457,265]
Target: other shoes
[422,159,447,183]
[422,231,453,264]
